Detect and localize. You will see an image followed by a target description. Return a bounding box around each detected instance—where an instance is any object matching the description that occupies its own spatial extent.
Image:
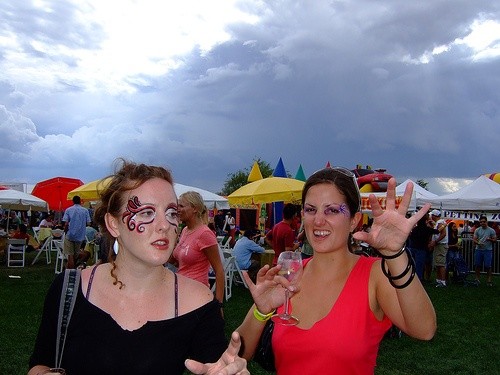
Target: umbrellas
[66,177,112,201]
[30,177,84,224]
[173,182,230,210]
[0,190,50,233]
[228,177,306,227]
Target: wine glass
[270,251,303,325]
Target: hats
[428,209,441,216]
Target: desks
[253,249,275,268]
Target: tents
[394,175,500,213]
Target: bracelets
[254,306,276,321]
[379,241,416,288]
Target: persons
[27,158,251,375]
[0,195,475,287]
[175,191,225,318]
[235,167,437,375]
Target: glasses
[313,166,362,211]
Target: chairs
[8,226,66,274]
[208,236,249,302]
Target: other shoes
[487,281,492,288]
[435,282,448,288]
[434,281,439,285]
[473,279,479,287]
